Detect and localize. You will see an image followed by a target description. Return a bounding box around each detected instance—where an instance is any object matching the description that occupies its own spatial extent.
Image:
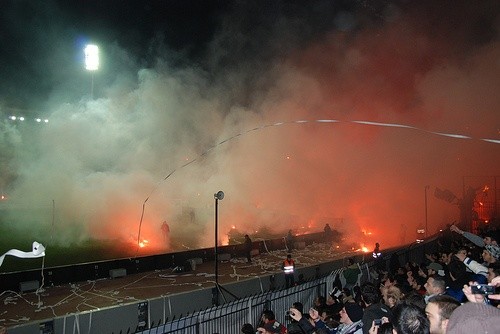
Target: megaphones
[214,191,225,200]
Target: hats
[426,263,443,271]
[324,316,343,328]
[446,302,500,334]
[485,242,500,258]
[328,287,343,303]
[345,304,363,323]
[241,323,256,334]
[413,272,426,284]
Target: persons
[282,255,296,289]
[415,231,424,244]
[241,323,256,334]
[287,302,315,334]
[161,221,170,243]
[288,229,294,252]
[399,223,406,243]
[188,207,196,223]
[446,302,500,334]
[314,282,393,334]
[309,302,364,334]
[244,235,252,263]
[257,327,273,334]
[257,310,287,334]
[424,295,461,334]
[324,224,331,242]
[462,275,500,309]
[369,303,430,334]
[369,221,500,311]
[372,243,381,260]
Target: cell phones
[256,330,260,334]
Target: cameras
[286,310,293,316]
[471,284,496,295]
[374,319,384,326]
